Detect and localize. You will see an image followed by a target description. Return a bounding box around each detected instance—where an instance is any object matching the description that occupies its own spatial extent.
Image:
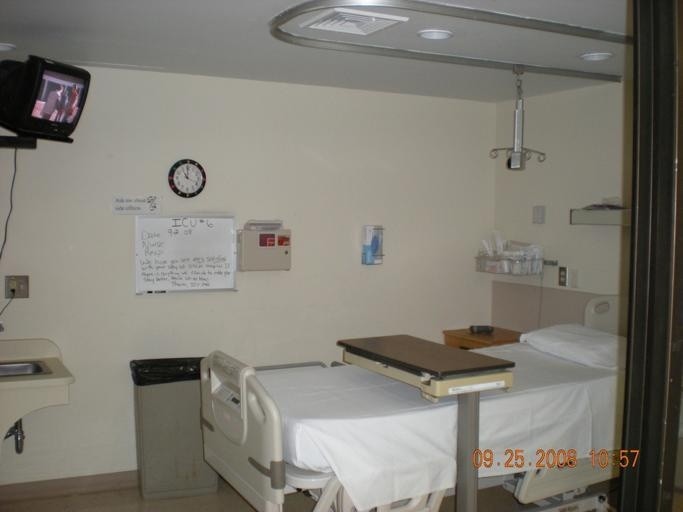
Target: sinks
[0,357,53,379]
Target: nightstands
[444,323,521,349]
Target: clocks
[168,158,207,198]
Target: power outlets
[5,276,30,299]
[559,267,567,287]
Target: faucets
[0,322,7,335]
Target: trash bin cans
[130,357,219,499]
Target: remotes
[470,325,494,333]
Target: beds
[198,294,621,512]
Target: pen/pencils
[147,291,167,294]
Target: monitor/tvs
[0,54,88,141]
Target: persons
[40,84,79,123]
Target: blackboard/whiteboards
[132,213,238,295]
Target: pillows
[521,322,627,370]
